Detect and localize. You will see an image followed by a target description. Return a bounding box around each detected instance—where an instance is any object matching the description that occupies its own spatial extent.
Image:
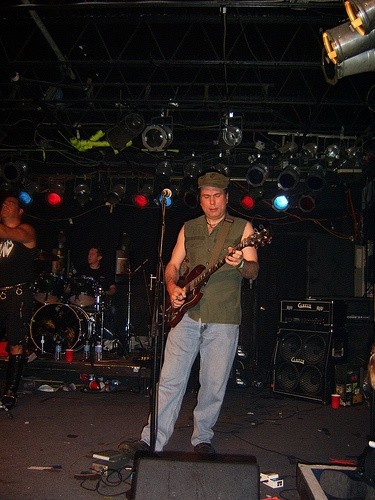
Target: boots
[0,353,25,411]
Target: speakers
[130,450,261,500]
[273,331,372,404]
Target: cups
[65,349,74,361]
[332,393,341,408]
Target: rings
[176,295,181,300]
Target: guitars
[164,224,273,328]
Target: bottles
[55,341,62,361]
[95,341,102,362]
[83,341,92,360]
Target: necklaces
[206,219,217,228]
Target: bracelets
[238,260,245,270]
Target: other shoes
[193,442,215,454]
[118,440,149,458]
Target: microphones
[162,188,172,198]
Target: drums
[28,302,92,355]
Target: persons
[119,173,259,459]
[0,197,37,410]
[75,248,116,297]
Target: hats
[197,171,230,188]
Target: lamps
[47,184,64,206]
[133,183,152,209]
[241,189,265,210]
[19,190,34,206]
[322,48,375,85]
[345,0,375,35]
[160,195,174,209]
[322,21,375,64]
[272,193,290,212]
